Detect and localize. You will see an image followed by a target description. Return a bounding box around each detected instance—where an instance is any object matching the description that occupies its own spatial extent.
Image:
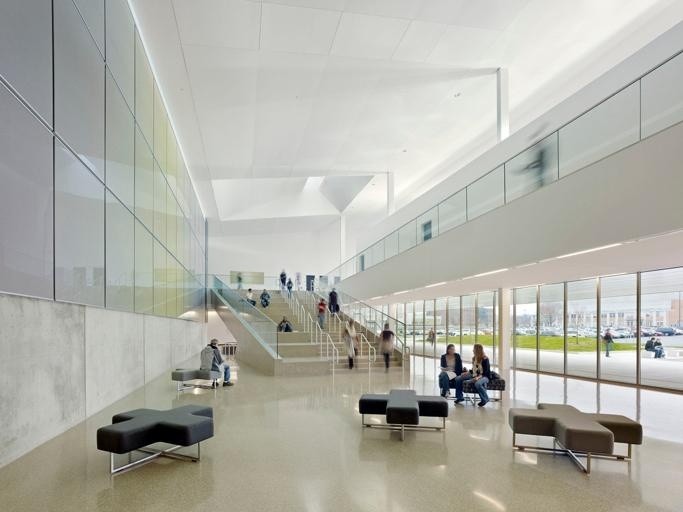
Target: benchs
[96,404,214,474]
[507,401,644,474]
[438,369,506,405]
[358,387,448,443]
[171,367,222,392]
[642,348,682,359]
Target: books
[441,368,456,380]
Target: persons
[604,329,613,357]
[644,337,659,358]
[259,289,270,308]
[280,269,285,289]
[343,319,359,369]
[379,323,394,369]
[237,272,242,288]
[286,278,292,298]
[246,288,256,306]
[318,299,326,329]
[654,339,663,358]
[428,328,434,346]
[438,343,462,397]
[206,339,234,386]
[453,343,489,406]
[328,288,336,314]
[278,316,292,332]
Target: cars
[397,324,682,339]
